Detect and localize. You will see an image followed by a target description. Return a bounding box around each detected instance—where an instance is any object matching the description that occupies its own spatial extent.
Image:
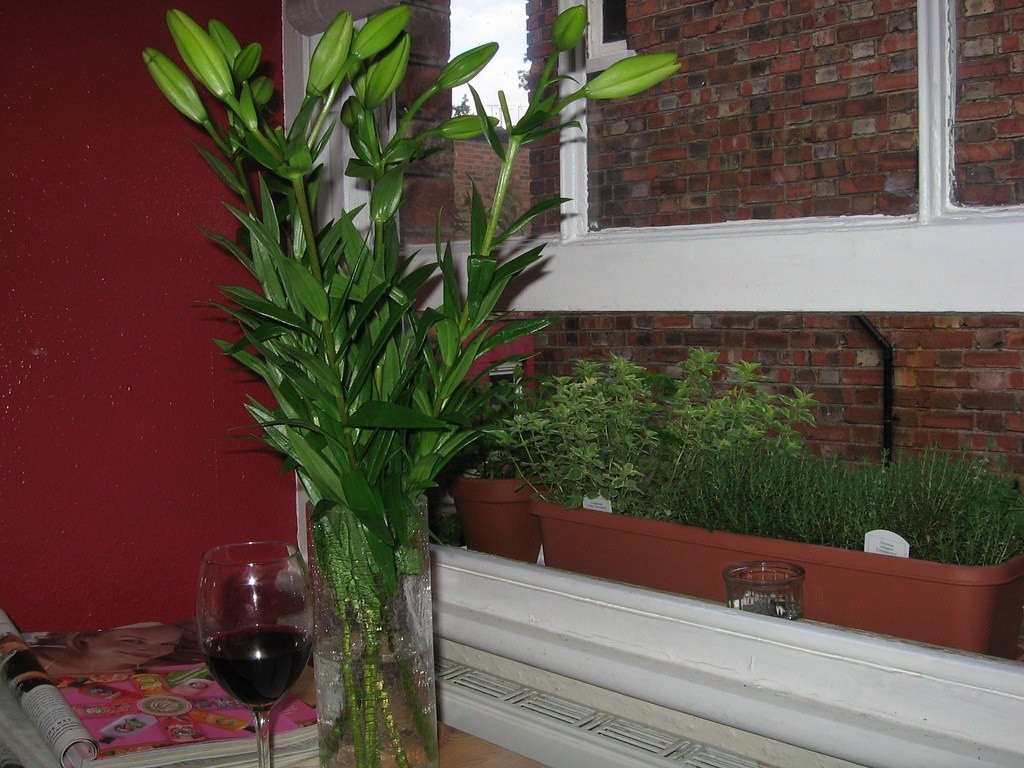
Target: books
[0,610,322,768]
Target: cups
[724,559,807,621]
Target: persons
[0,615,206,697]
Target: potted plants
[483,351,1024,655]
[448,365,552,564]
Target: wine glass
[197,540,316,768]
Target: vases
[310,498,438,768]
